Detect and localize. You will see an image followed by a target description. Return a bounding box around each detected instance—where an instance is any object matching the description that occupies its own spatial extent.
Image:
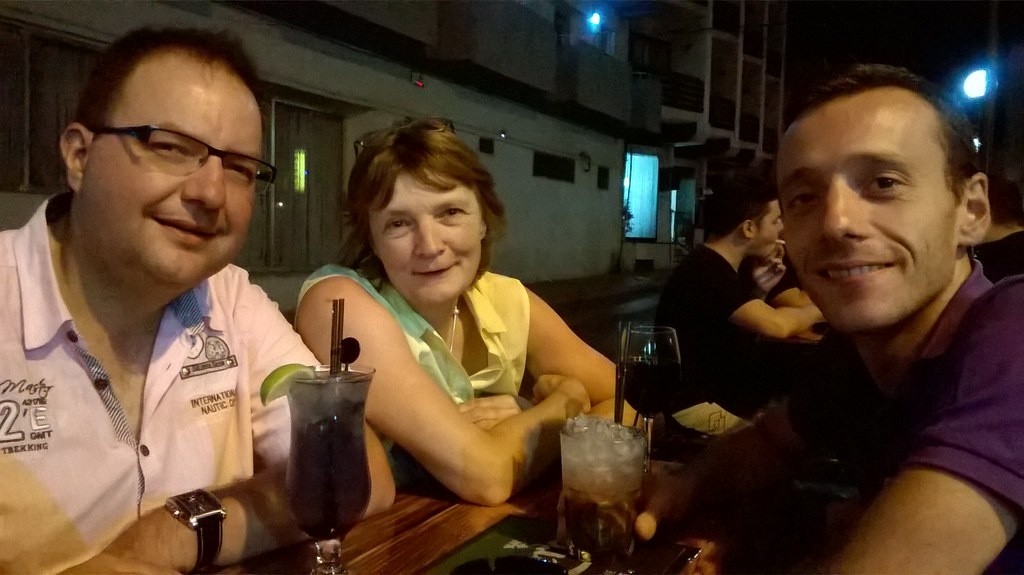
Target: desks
[214,389,807,575]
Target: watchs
[164,488,229,573]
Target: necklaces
[446,301,460,352]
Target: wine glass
[619,324,681,480]
[284,371,373,575]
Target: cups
[559,426,648,569]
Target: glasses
[90,125,278,196]
[353,116,455,162]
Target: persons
[294,117,645,506]
[654,170,824,439]
[968,173,1024,285]
[557,61,1024,575]
[0,25,395,575]
